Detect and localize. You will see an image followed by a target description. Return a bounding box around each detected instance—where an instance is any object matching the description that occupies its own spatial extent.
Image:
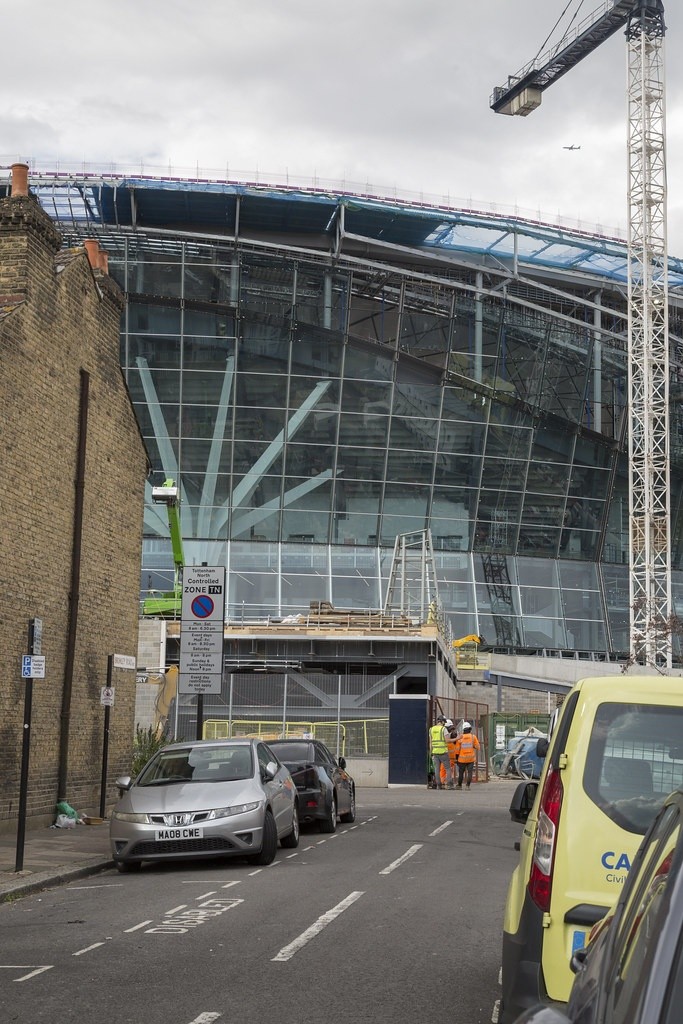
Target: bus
[108,737,300,874]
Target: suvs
[506,782,682,1024]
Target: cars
[263,738,356,833]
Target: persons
[429,715,463,789]
[451,722,480,791]
[440,719,457,790]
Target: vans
[494,675,683,1024]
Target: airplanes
[562,144,582,151]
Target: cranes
[488,0,677,667]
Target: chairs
[596,757,652,816]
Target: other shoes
[465,786,470,791]
[437,784,441,790]
[442,784,446,789]
[446,783,454,790]
[455,785,462,790]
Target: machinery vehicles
[138,478,188,620]
[450,634,488,671]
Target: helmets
[444,719,454,727]
[438,715,447,723]
[462,722,471,730]
